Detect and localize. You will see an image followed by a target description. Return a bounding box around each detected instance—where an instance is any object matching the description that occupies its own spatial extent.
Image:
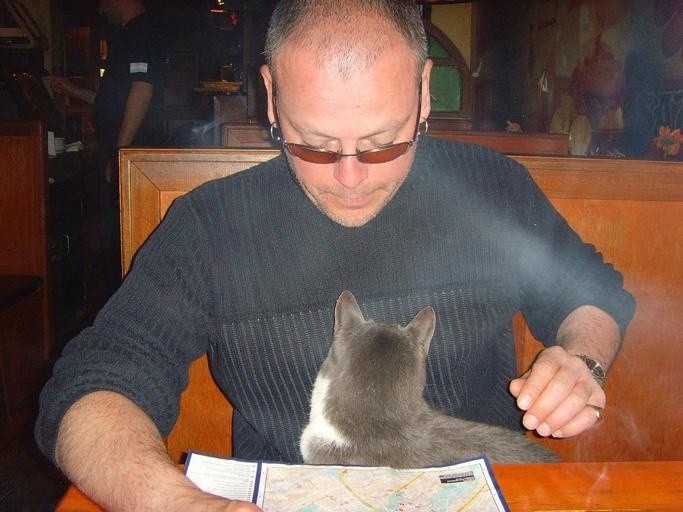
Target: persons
[34,0,636,512]
[40,0,176,185]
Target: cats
[293,287,566,470]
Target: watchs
[574,355,608,389]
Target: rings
[586,404,602,418]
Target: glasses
[271,76,424,165]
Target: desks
[51,451,683,510]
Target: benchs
[221,124,569,156]
[119,149,683,459]
